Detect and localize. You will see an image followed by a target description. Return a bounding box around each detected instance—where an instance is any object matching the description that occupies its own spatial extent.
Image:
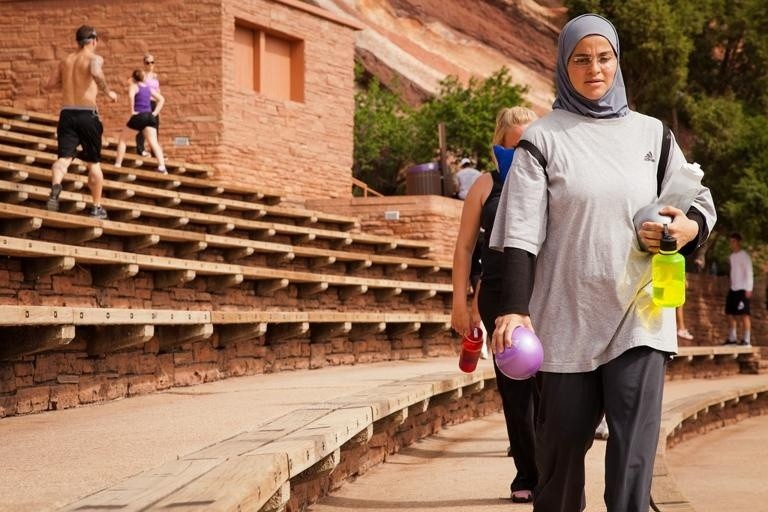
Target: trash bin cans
[406,162,443,195]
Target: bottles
[457,327,484,373]
[650,223,686,309]
[657,161,706,215]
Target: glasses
[572,55,612,67]
[145,60,155,64]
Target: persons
[451,105,539,504]
[47,25,118,219]
[489,12,719,512]
[725,233,754,346]
[452,157,482,200]
[114,68,168,175]
[127,53,160,157]
[676,306,695,340]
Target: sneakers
[46,183,63,212]
[722,339,753,347]
[512,490,533,504]
[677,328,694,341]
[115,148,168,176]
[89,206,108,218]
